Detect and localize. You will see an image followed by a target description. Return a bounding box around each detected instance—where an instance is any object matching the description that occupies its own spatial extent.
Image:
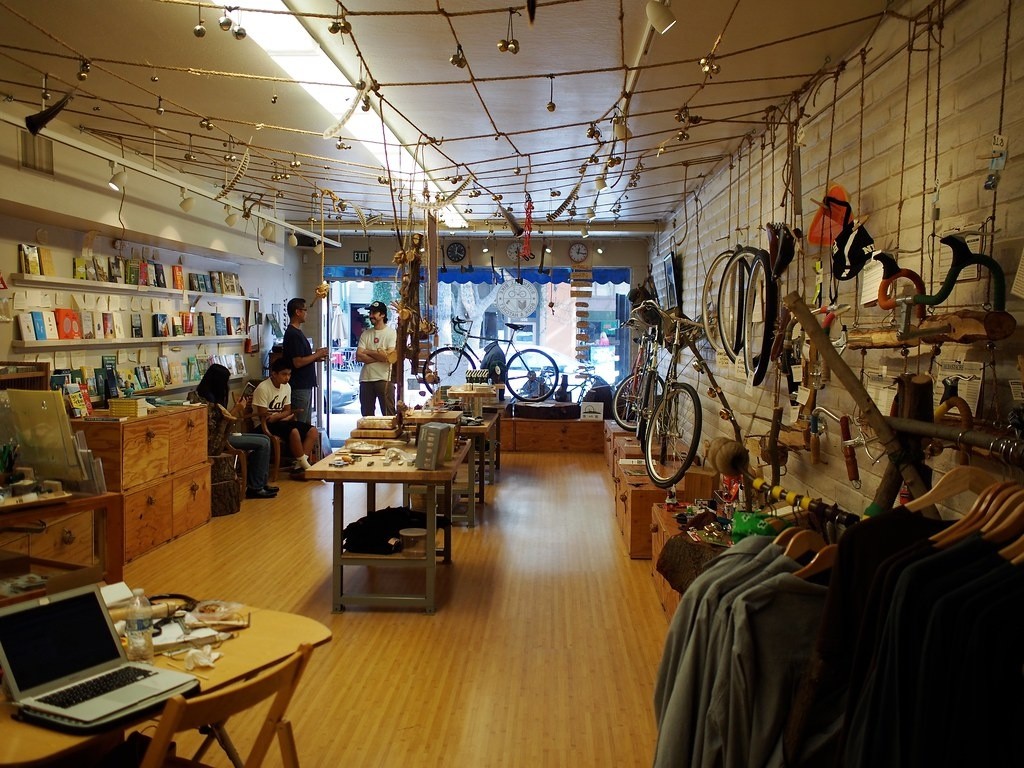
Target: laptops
[0,583,198,723]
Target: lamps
[580,225,590,239]
[314,239,323,256]
[177,187,196,213]
[480,242,490,253]
[289,231,298,246]
[586,208,594,220]
[645,0,676,35]
[613,120,632,143]
[213,0,470,228]
[597,245,607,255]
[261,218,275,241]
[546,244,554,254]
[109,161,129,191]
[596,175,608,191]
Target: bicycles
[631,300,706,489]
[422,316,559,402]
[612,318,665,432]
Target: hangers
[907,419,1024,566]
[752,490,851,580]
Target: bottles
[125,588,154,668]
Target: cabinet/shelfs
[11,271,251,397]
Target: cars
[323,370,360,412]
[468,342,598,386]
[331,347,364,371]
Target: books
[53,349,247,418]
[18,243,244,297]
[143,609,222,655]
[16,305,249,341]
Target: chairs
[139,637,314,768]
[340,350,355,371]
[337,349,344,352]
[233,388,295,482]
[225,405,253,498]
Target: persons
[356,300,397,415]
[250,357,323,481]
[283,298,329,423]
[195,363,279,498]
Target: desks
[396,409,504,508]
[0,488,125,606]
[0,595,336,768]
[305,434,474,616]
[332,351,344,369]
[458,394,514,486]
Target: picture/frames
[662,251,677,309]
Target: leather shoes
[245,484,279,499]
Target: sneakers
[289,467,323,481]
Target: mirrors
[247,296,261,354]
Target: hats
[365,301,387,312]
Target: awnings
[322,265,630,285]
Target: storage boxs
[171,461,215,542]
[602,418,737,630]
[0,523,28,556]
[31,506,95,574]
[98,475,173,568]
[68,411,170,491]
[151,401,211,476]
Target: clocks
[506,243,524,262]
[569,243,589,263]
[446,242,466,262]
